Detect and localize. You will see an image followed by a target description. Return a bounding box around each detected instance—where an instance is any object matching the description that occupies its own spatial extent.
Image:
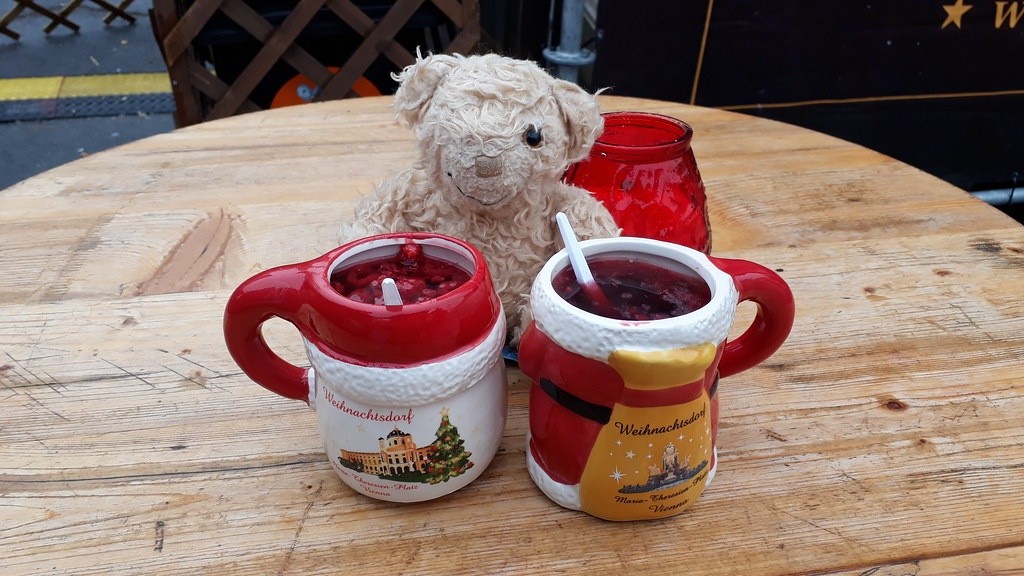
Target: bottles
[563,112,711,258]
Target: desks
[0,92,1024,576]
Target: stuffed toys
[330,45,624,352]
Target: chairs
[149,0,510,128]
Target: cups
[224,233,508,502]
[518,237,796,522]
